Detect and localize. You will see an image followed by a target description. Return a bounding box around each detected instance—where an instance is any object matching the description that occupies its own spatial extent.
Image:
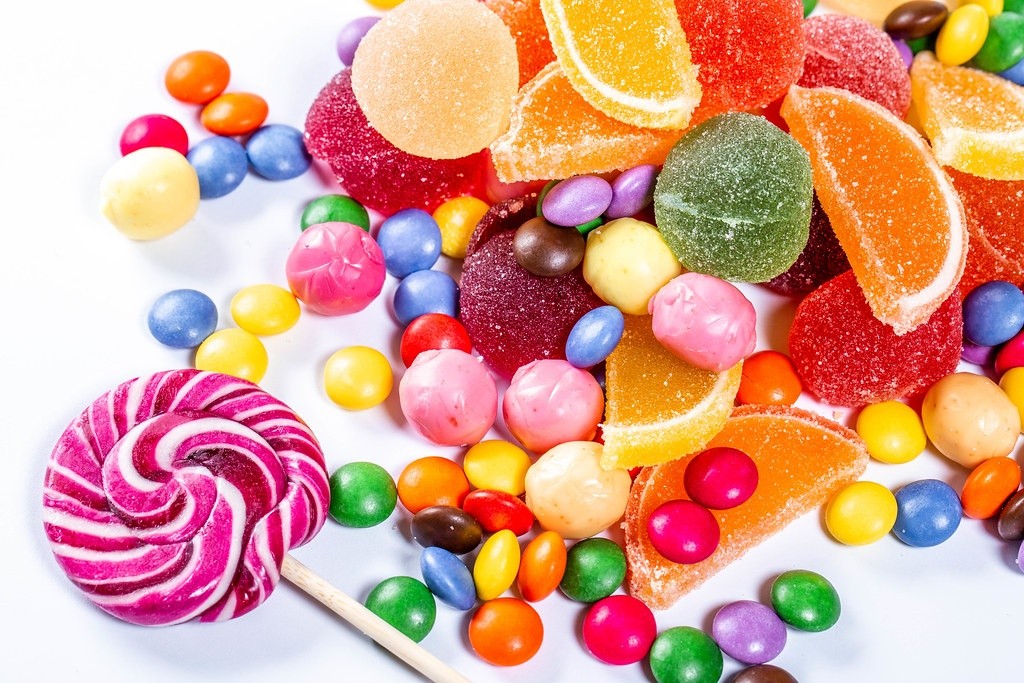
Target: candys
[40,0,1024,683]
